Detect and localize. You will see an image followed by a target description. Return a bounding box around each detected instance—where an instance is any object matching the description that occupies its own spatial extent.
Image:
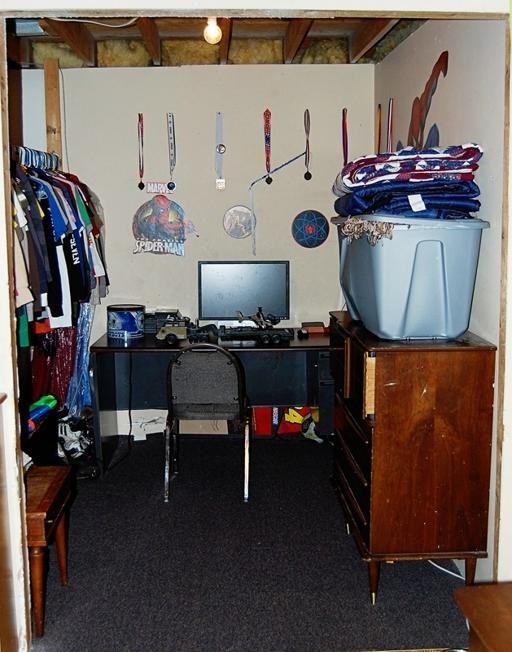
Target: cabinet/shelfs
[329,310,497,605]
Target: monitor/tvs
[198,261,290,321]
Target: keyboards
[219,325,295,341]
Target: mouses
[297,328,309,339]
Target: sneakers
[57,407,94,460]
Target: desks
[90,327,345,480]
[451,583,512,652]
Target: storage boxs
[330,215,491,342]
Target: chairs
[163,344,254,502]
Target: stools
[24,465,78,638]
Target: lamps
[203,19,223,44]
